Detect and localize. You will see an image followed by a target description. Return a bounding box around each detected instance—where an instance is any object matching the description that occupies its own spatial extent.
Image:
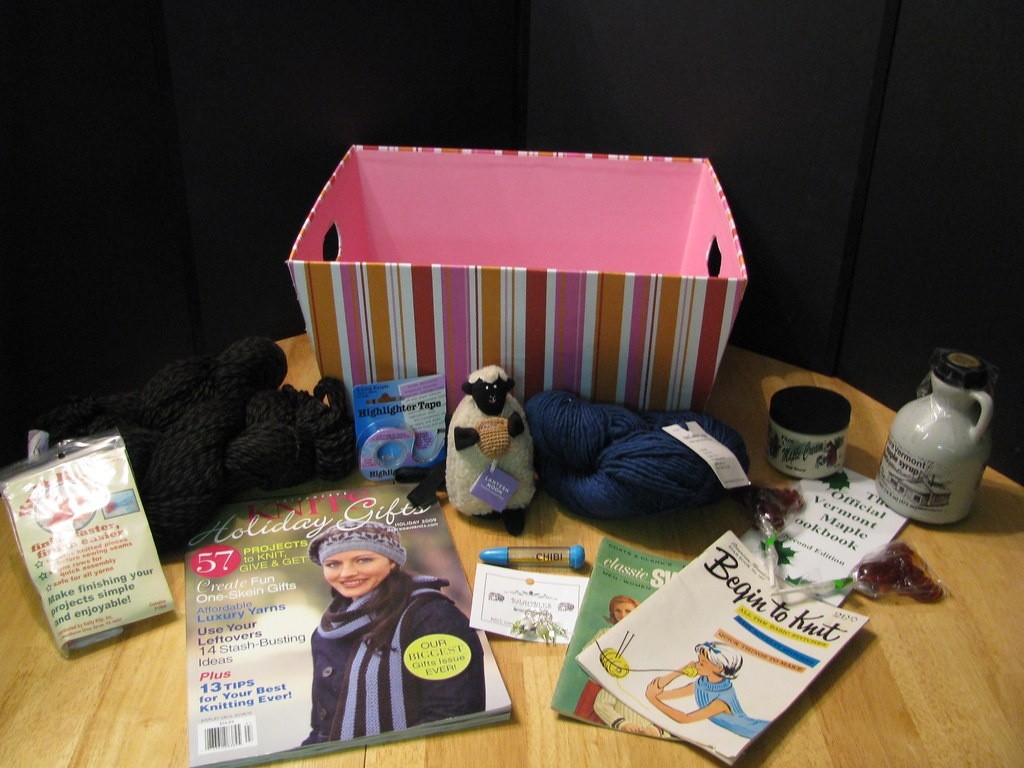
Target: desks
[0,330,1024,768]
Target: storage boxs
[285,144,749,426]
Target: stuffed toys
[443,363,538,537]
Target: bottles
[876,352,996,521]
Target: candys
[739,486,806,589]
[771,543,942,603]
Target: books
[548,533,870,768]
[181,482,512,768]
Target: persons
[300,521,486,747]
[574,595,662,728]
